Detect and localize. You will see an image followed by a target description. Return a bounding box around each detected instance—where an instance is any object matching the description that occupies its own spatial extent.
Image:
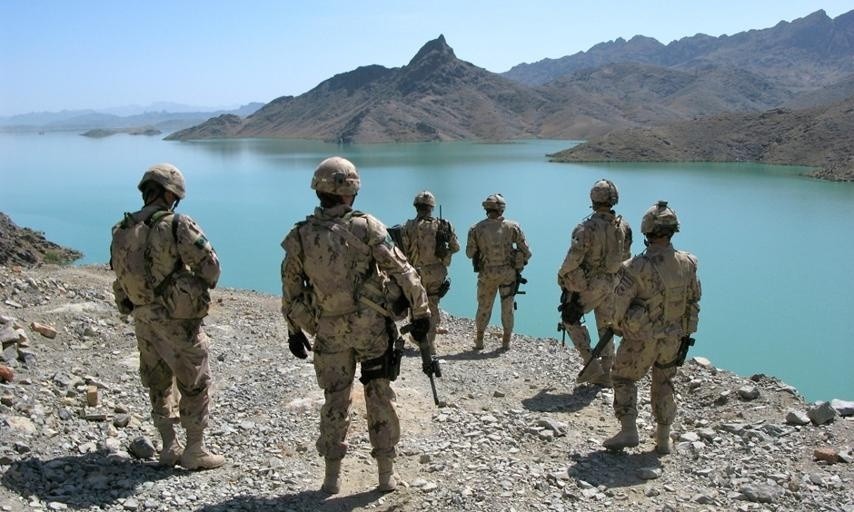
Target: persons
[280,157,431,494]
[466,194,532,351]
[110,165,225,470]
[603,202,701,453]
[400,192,460,354]
[559,179,632,388]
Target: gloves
[288,330,311,359]
[412,318,429,340]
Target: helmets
[310,157,361,197]
[641,200,679,235]
[413,191,435,207]
[482,193,505,216]
[137,163,187,200]
[590,179,619,206]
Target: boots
[591,356,613,388]
[324,458,341,494]
[657,424,670,455]
[376,456,397,492]
[477,332,484,349]
[503,329,512,350]
[576,349,604,383]
[181,429,226,470]
[160,424,185,466]
[602,414,639,446]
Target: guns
[579,328,613,378]
[557,287,570,347]
[386,225,442,405]
